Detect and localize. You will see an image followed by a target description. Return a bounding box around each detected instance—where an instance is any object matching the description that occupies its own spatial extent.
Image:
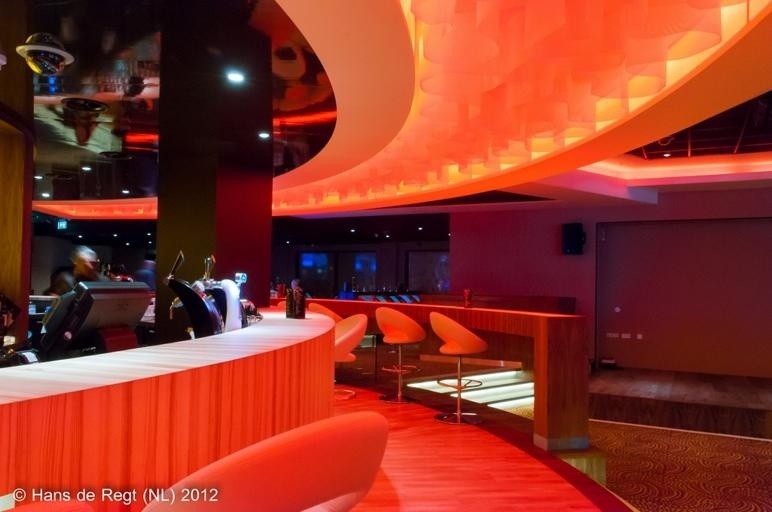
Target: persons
[56,248,109,293]
[286,279,304,319]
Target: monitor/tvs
[41,282,152,360]
[28,302,36,314]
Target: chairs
[139,411,386,512]
[335,314,367,363]
[430,310,488,424]
[375,307,425,403]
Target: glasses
[84,259,101,267]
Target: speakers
[562,223,583,254]
